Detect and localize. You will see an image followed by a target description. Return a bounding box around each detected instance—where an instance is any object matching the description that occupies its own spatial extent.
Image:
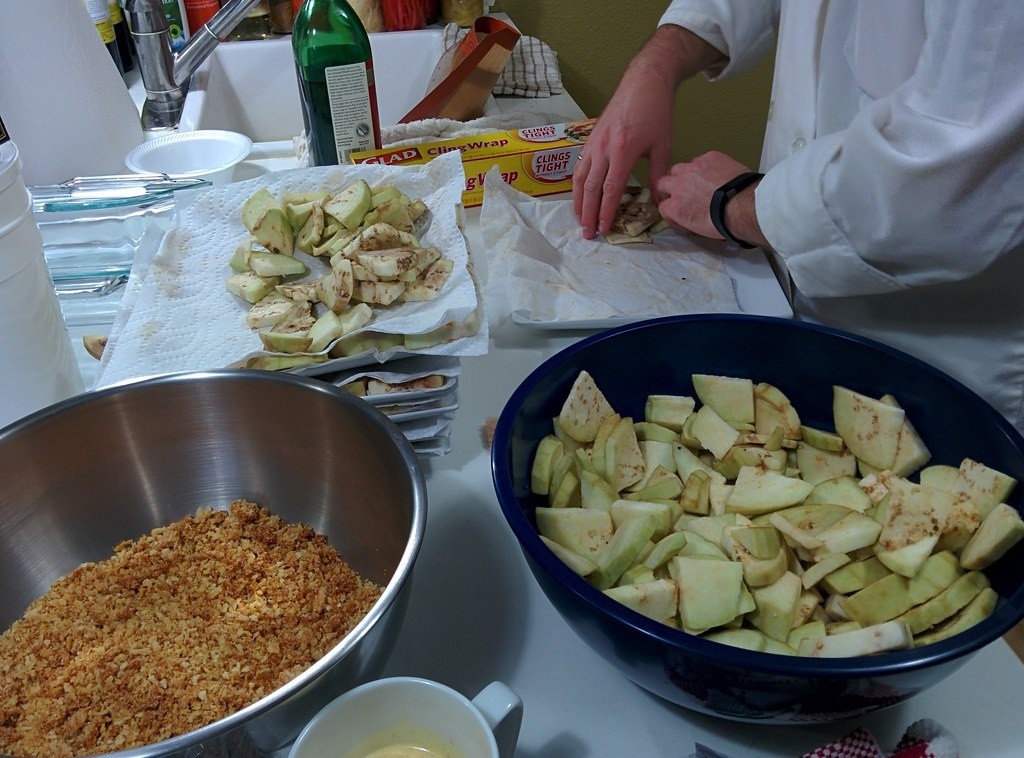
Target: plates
[509,244,794,331]
[296,349,459,460]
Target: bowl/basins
[0,365,429,758]
[123,129,254,187]
[490,312,1024,729]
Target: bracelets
[709,173,765,249]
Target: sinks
[179,27,445,160]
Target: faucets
[124,0,262,132]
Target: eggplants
[83,335,108,360]
[531,370,1024,659]
[611,199,663,238]
[224,178,479,407]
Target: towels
[440,22,564,98]
[290,109,577,169]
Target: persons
[573,1,1023,435]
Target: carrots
[380,0,438,31]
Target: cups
[184,0,219,36]
[221,0,273,40]
[0,118,88,431]
[439,0,484,29]
[288,676,524,758]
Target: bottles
[158,0,190,53]
[290,0,383,168]
[83,0,125,78]
[108,0,136,73]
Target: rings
[578,155,582,160]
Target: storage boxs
[349,117,598,207]
